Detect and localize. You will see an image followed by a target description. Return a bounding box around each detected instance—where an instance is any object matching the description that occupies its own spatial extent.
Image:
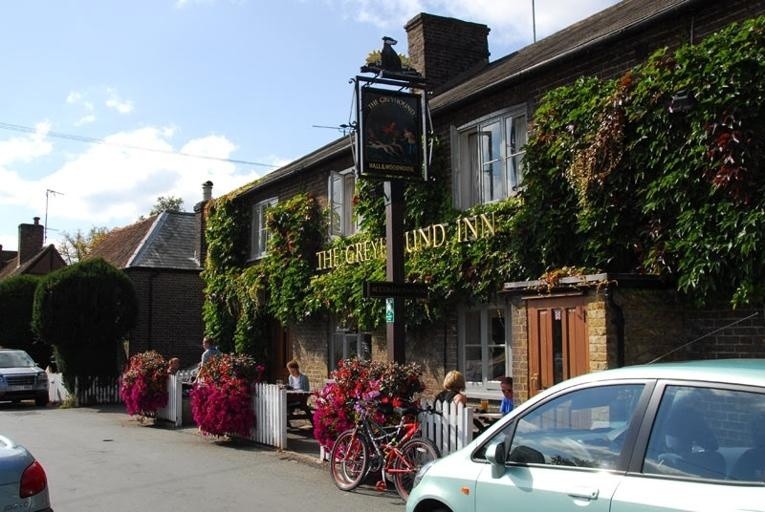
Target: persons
[167,357,182,375]
[499,378,512,414]
[433,370,467,417]
[286,361,309,409]
[193,336,221,385]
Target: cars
[407,358,765,512]
[0,349,49,406]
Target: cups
[480,398,489,411]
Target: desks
[287,390,315,429]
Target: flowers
[310,352,429,468]
[187,350,266,440]
[117,349,172,418]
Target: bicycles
[329,398,441,503]
